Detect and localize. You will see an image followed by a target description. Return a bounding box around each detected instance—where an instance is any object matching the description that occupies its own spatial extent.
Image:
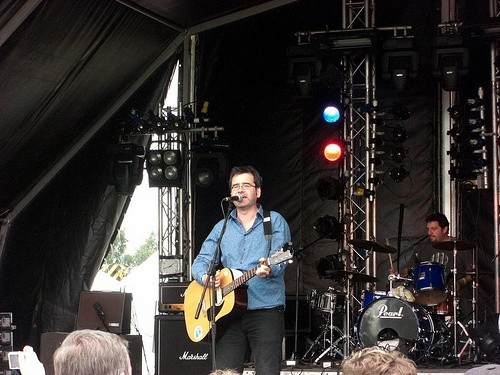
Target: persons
[54,330,132,375]
[389,213,472,291]
[342,346,417,375]
[18,346,45,375]
[191,166,291,375]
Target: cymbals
[348,239,397,254]
[431,239,477,252]
[325,268,381,283]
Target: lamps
[291,26,420,277]
[428,19,470,91]
[447,98,487,180]
[117,103,228,191]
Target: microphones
[223,194,242,201]
[93,303,105,315]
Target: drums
[386,277,416,295]
[411,260,450,308]
[436,278,461,316]
[309,289,353,313]
[355,296,435,364]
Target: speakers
[154,314,213,375]
[39,332,142,375]
[76,291,133,334]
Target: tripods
[300,283,361,363]
[433,244,484,366]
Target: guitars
[184,240,295,345]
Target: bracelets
[463,278,466,284]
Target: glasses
[231,183,256,190]
